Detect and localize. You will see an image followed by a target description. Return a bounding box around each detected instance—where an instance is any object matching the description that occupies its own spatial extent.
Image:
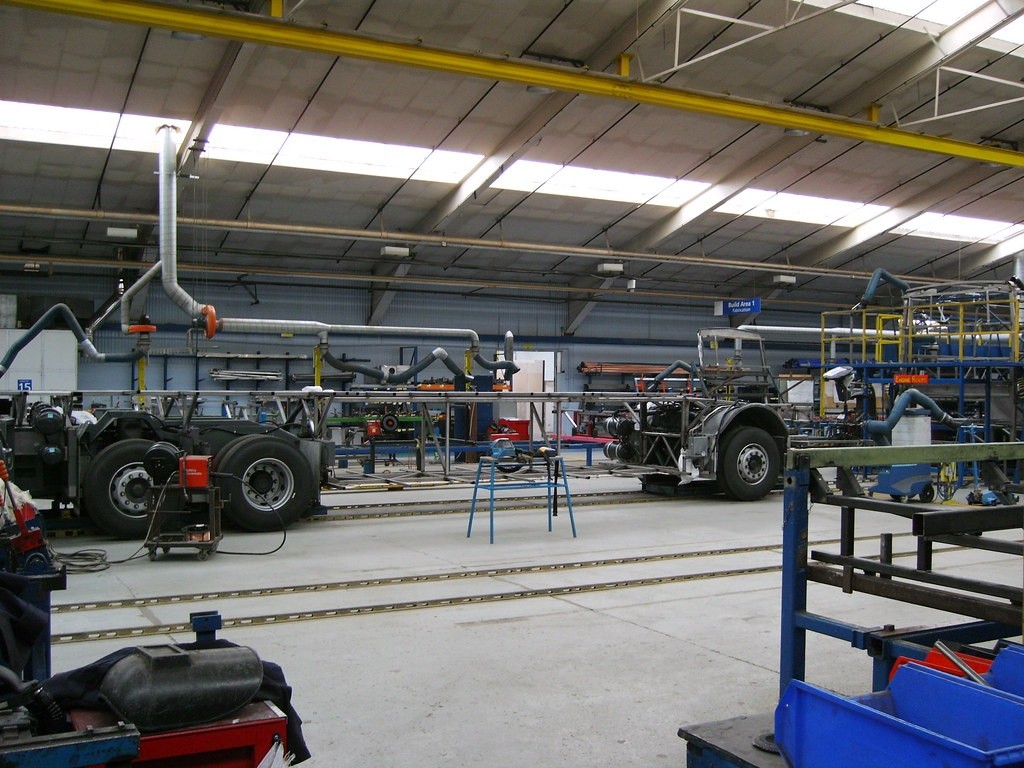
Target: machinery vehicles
[0,393,336,533]
[603,329,877,502]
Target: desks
[465,455,576,546]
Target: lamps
[773,272,796,284]
[380,244,409,258]
[627,261,635,292]
[107,223,138,239]
[597,261,623,273]
[441,232,448,247]
